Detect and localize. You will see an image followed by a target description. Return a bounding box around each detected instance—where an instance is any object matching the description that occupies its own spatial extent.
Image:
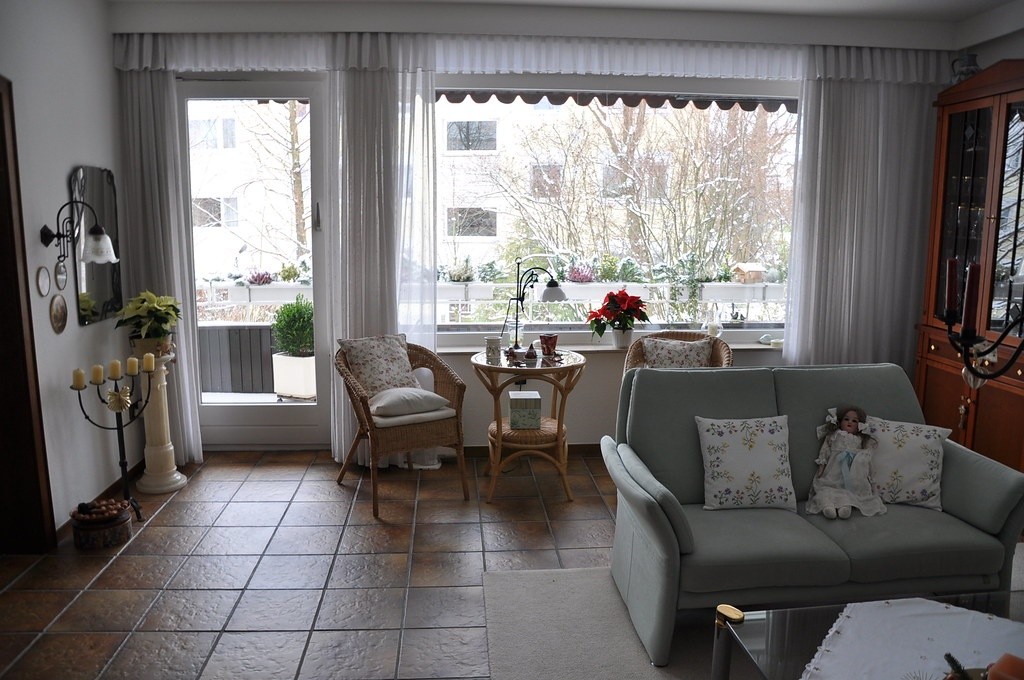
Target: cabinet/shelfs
[913,58,1024,473]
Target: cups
[484,335,502,358]
[540,333,558,355]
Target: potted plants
[270,292,317,399]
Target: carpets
[481,543,1024,680]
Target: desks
[470,347,587,503]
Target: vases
[612,327,633,349]
[127,333,173,359]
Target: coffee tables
[709,590,1024,680]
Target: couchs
[599,362,1024,668]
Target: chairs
[623,331,733,377]
[334,333,470,518]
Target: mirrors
[67,165,125,328]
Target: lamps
[500,256,570,357]
[39,202,119,265]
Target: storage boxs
[508,391,542,430]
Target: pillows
[867,414,953,512]
[695,415,797,514]
[367,387,451,416]
[336,334,422,398]
[641,336,716,369]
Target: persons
[805,404,887,519]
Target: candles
[126,356,140,376]
[962,263,981,328]
[143,353,155,371]
[109,359,122,379]
[72,367,86,388]
[701,309,723,338]
[91,363,104,384]
[945,258,957,310]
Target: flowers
[585,287,653,342]
[113,289,183,341]
[79,292,100,326]
